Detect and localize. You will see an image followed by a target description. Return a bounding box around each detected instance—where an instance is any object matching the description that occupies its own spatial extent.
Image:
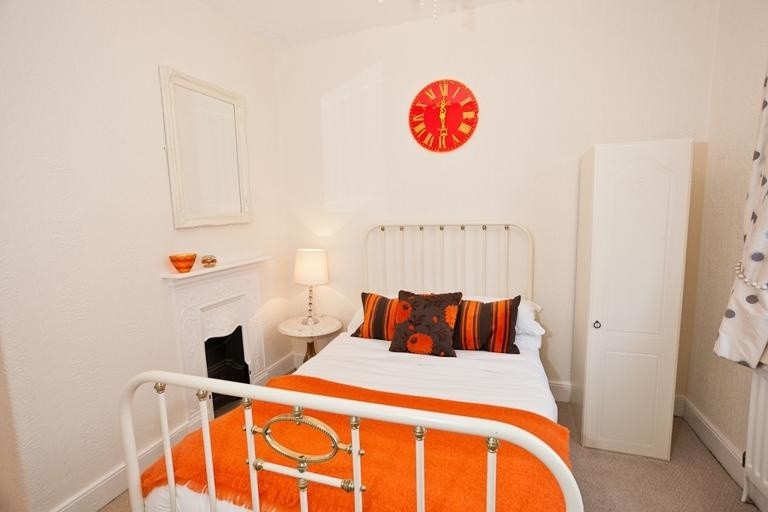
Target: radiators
[740,362,768,505]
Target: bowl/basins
[169,253,198,274]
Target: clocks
[408,78,480,153]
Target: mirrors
[158,63,253,228]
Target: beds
[117,223,589,512]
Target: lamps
[291,250,329,326]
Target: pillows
[348,287,547,358]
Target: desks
[275,316,342,372]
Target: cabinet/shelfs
[572,141,694,461]
